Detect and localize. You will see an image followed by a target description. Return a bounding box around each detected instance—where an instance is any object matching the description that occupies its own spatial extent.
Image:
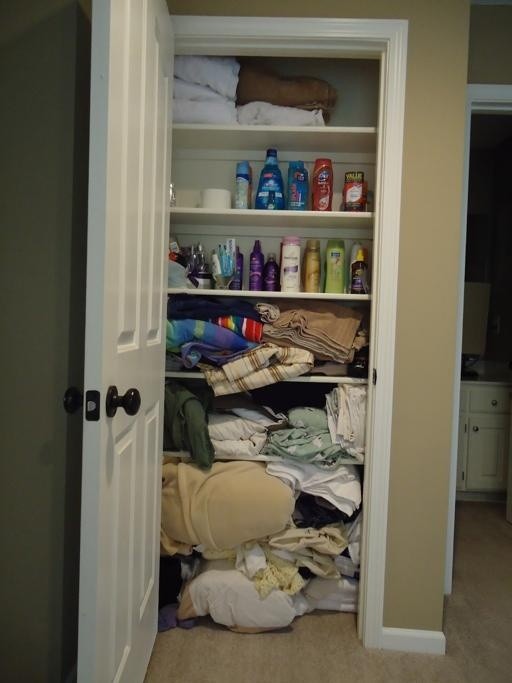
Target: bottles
[248,239,264,290]
[348,248,367,294]
[261,252,279,292]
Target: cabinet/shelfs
[462,280,492,355]
[460,381,512,492]
[162,122,380,466]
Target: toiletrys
[324,238,345,294]
[349,240,363,292]
[286,159,310,210]
[280,236,301,291]
[263,252,280,291]
[312,159,334,212]
[303,237,322,292]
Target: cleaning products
[351,251,368,293]
